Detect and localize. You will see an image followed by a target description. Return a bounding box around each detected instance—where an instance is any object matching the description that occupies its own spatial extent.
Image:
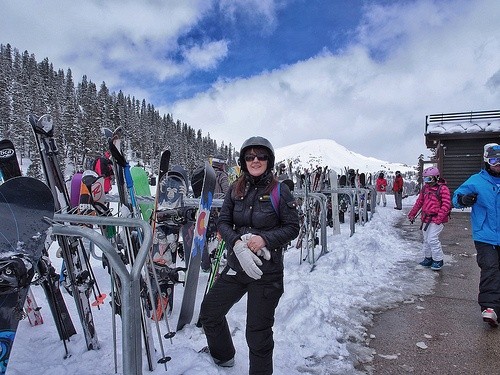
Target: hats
[483,143,500,158]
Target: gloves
[458,193,478,208]
[241,233,271,260]
[233,241,263,280]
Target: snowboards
[60,169,116,297]
[338,165,372,226]
[130,165,154,222]
[144,160,217,335]
[0,175,56,375]
[329,168,341,235]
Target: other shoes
[431,260,444,270]
[212,358,235,367]
[419,258,432,268]
[394,207,402,210]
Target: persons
[211,154,230,195]
[452,143,500,328]
[376,173,388,207]
[391,171,403,210]
[94,148,115,194]
[408,167,451,270]
[200,136,300,375]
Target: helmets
[239,137,275,170]
[422,167,440,180]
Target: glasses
[423,177,433,183]
[484,157,500,166]
[244,153,268,161]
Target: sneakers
[482,308,499,327]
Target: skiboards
[1,111,100,352]
[289,160,328,249]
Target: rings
[252,246,255,249]
